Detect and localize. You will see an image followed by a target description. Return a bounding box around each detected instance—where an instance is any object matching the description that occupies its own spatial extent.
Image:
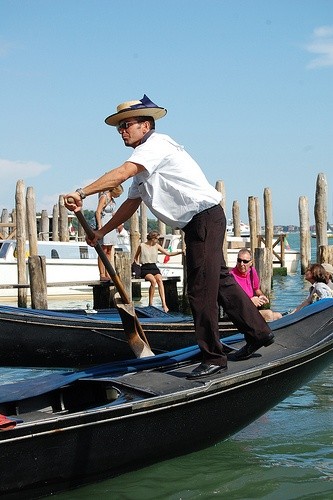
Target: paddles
[67,196,155,358]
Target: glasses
[237,258,250,263]
[117,121,139,134]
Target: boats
[0,236,168,301]
[0,304,291,371]
[0,295,333,500]
[311,228,333,238]
[152,232,301,276]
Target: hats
[321,264,333,273]
[105,94,167,126]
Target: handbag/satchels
[87,211,105,230]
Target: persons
[289,263,333,316]
[229,249,282,321]
[94,184,124,281]
[115,223,131,254]
[63,95,274,380]
[134,231,185,313]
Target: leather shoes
[230,333,275,360]
[186,358,227,380]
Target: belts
[195,204,220,217]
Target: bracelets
[76,188,85,199]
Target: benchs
[0,276,181,312]
